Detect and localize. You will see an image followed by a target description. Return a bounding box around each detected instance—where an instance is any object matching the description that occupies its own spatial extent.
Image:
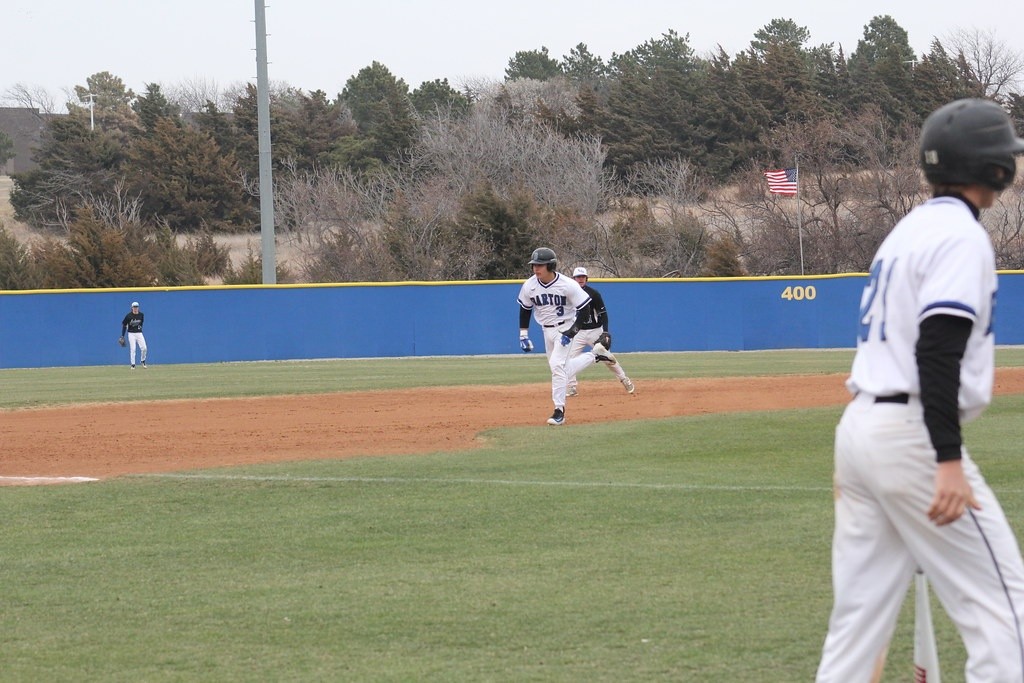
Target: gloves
[560,333,571,346]
[519,330,534,352]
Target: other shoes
[140,361,147,369]
[131,364,135,369]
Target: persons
[815,99,1024,683]
[517,247,617,425]
[119,302,147,370]
[565,267,634,397]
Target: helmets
[132,302,140,307]
[919,98,1024,189]
[527,248,557,264]
[572,267,587,277]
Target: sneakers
[549,405,565,425]
[621,377,634,393]
[591,343,616,365]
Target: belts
[872,393,910,404]
[544,321,564,327]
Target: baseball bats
[913,566,941,683]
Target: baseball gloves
[119,338,126,347]
[593,333,611,351]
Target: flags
[763,169,798,195]
